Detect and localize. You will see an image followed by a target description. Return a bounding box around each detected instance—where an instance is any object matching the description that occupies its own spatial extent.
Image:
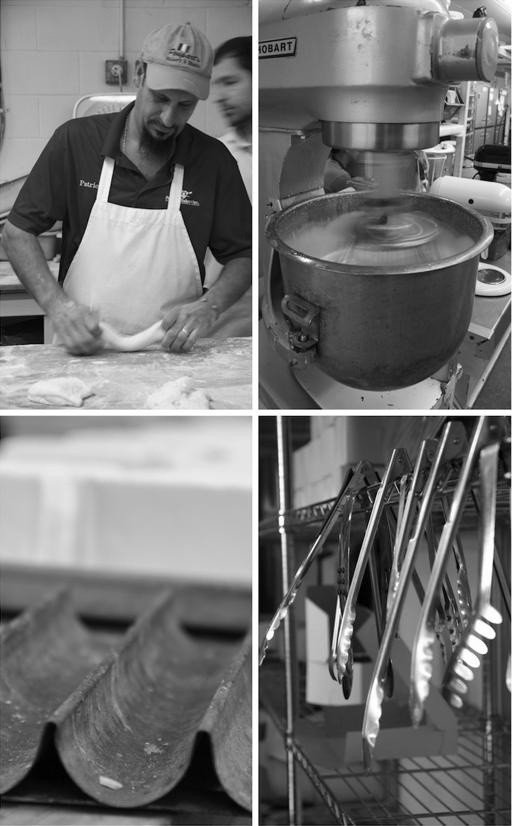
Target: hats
[139,21,213,100]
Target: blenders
[259,1,500,410]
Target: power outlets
[105,60,127,83]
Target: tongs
[259,416,511,777]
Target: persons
[0,22,252,356]
[321,148,431,193]
[210,37,251,203]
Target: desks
[0,260,60,317]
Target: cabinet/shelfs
[259,417,511,826]
